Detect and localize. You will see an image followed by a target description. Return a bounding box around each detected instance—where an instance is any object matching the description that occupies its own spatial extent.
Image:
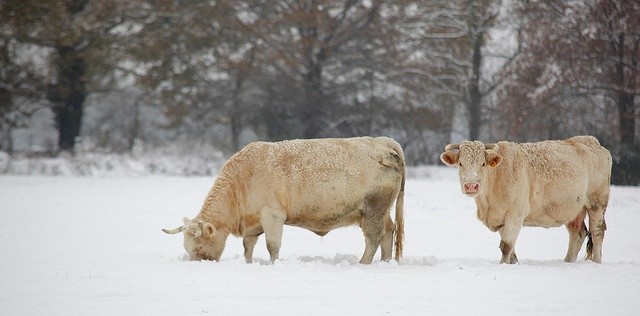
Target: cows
[162,136,405,263]
[441,136,612,264]
